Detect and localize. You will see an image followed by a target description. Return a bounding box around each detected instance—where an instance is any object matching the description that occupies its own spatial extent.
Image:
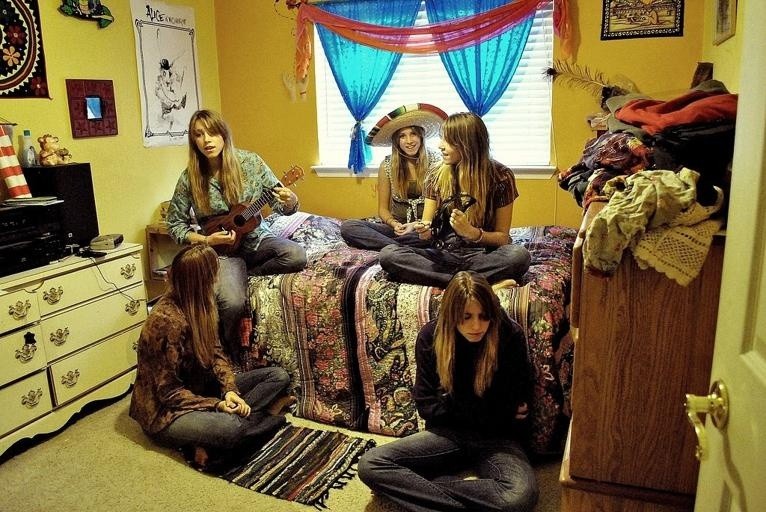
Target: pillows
[263,210,343,267]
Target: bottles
[422,194,439,223]
[21,130,36,168]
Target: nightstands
[146,224,202,306]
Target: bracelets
[473,228,484,243]
[203,236,208,246]
[389,219,398,226]
[385,216,393,223]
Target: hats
[364,103,450,148]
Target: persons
[129,243,298,476]
[358,270,541,511]
[379,112,531,285]
[167,109,306,346]
[341,104,449,250]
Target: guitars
[205,166,304,253]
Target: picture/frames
[65,78,118,138]
[601,0,685,40]
[712,0,736,45]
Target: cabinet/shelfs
[0,241,148,456]
[559,197,726,508]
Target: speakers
[21,163,99,250]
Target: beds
[237,218,580,466]
